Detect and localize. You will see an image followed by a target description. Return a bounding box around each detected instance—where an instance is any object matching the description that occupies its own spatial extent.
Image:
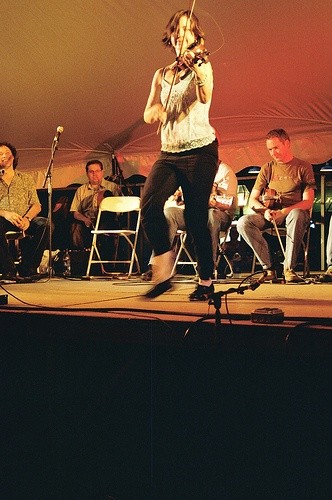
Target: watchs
[24,216,31,222]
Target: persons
[70,160,125,275]
[236,128,316,284]
[142,160,236,281]
[0,142,54,282]
[142,9,220,301]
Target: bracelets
[195,75,208,86]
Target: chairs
[5,196,313,282]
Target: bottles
[63,249,72,276]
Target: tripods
[15,139,91,284]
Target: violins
[258,188,282,208]
[172,36,210,73]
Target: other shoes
[257,268,277,283]
[144,279,173,299]
[141,270,153,280]
[282,268,298,282]
[318,265,332,282]
[28,272,48,279]
[188,282,215,301]
[0,269,17,280]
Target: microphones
[54,126,63,141]
[250,273,269,291]
